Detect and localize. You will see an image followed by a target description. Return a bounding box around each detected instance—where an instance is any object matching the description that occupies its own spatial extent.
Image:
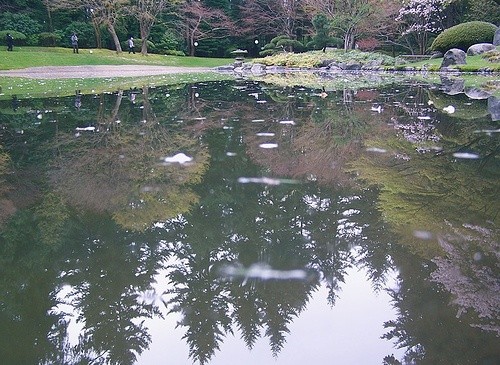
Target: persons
[129,36,136,54]
[5,33,14,51]
[72,33,79,54]
